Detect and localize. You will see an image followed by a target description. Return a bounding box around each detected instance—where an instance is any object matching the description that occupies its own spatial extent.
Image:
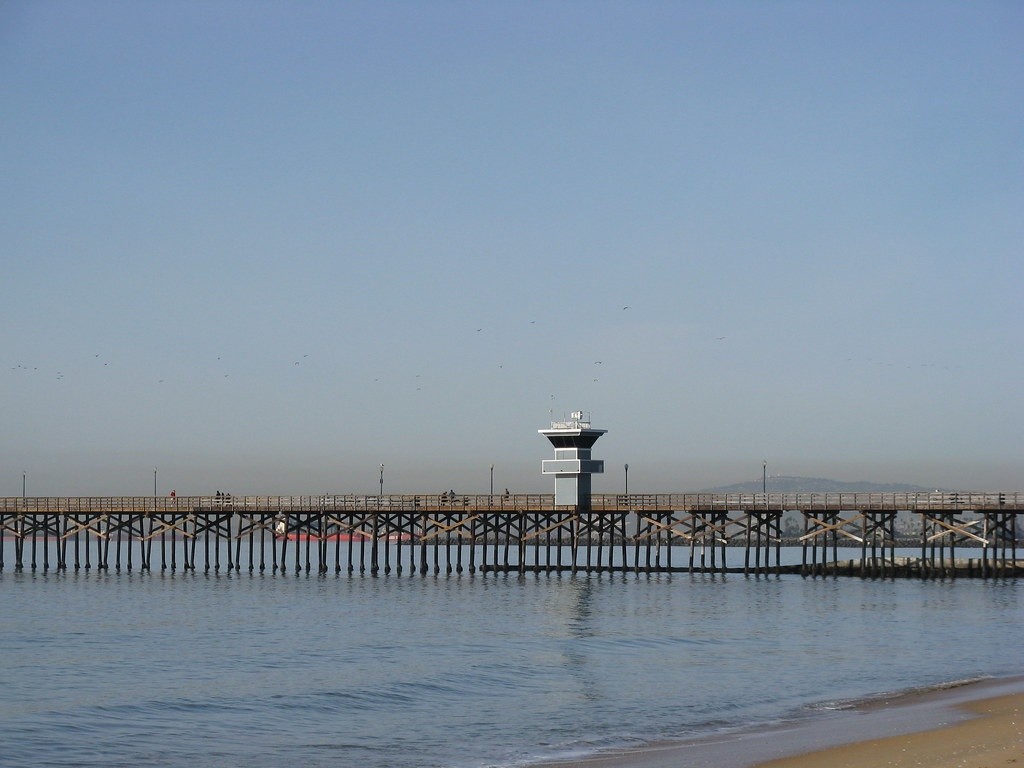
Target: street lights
[152,465,158,512]
[378,463,385,506]
[763,460,767,505]
[489,462,496,506]
[624,463,629,506]
[22,470,27,508]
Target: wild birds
[623,304,628,311]
[95,352,111,369]
[592,378,600,381]
[10,362,66,381]
[156,349,309,383]
[375,372,422,392]
[715,335,726,341]
[595,360,605,365]
[475,319,556,415]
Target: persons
[440,488,510,506]
[170,490,176,507]
[216,491,231,507]
